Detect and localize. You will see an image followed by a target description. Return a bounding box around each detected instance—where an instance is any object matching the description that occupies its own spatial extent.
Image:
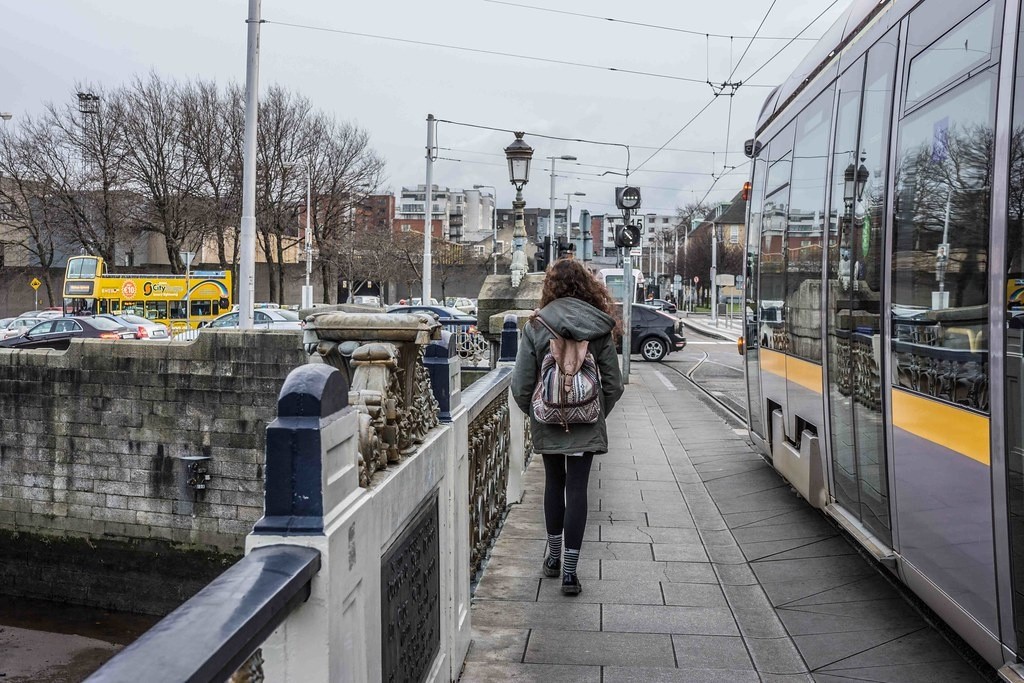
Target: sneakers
[561,573,582,593]
[543,557,561,576]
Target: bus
[596,269,646,302]
[739,0,1024,683]
[61,256,232,333]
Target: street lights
[692,218,716,320]
[564,193,586,252]
[504,133,534,273]
[547,154,577,264]
[939,186,991,310]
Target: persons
[511,260,625,596]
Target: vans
[346,296,385,306]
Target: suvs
[604,301,687,362]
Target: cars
[396,297,478,317]
[387,307,478,354]
[172,308,303,343]
[646,299,677,315]
[0,306,171,351]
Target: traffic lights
[615,225,641,248]
[535,236,551,270]
[558,237,573,259]
[615,186,641,210]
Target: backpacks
[531,316,603,431]
